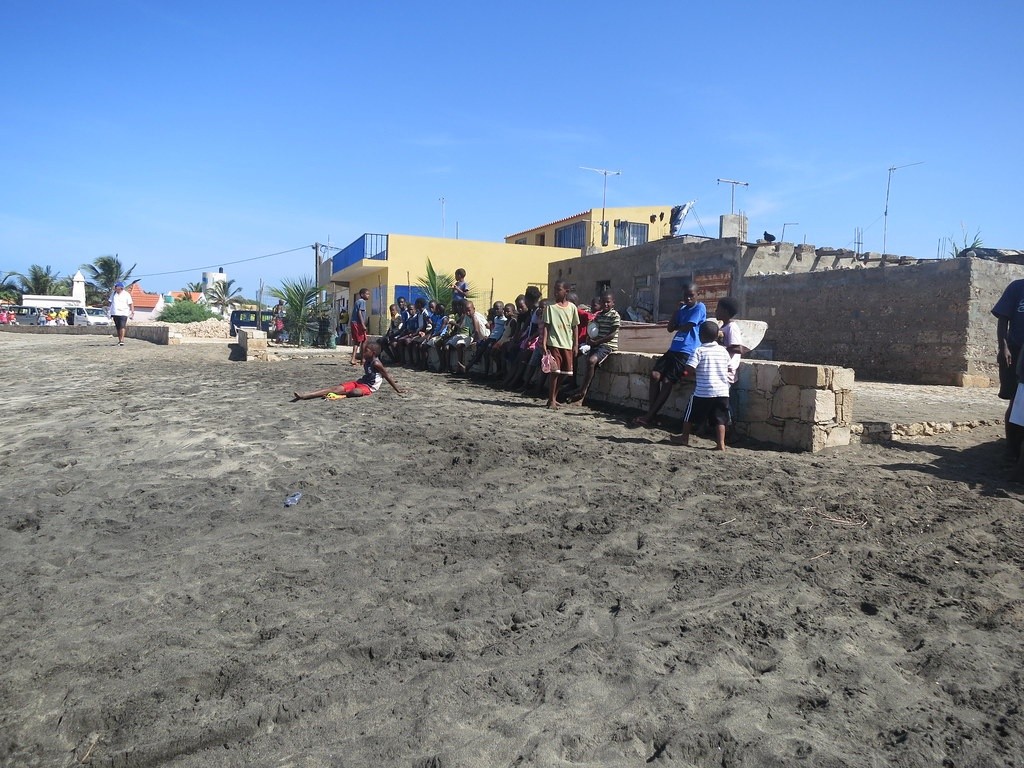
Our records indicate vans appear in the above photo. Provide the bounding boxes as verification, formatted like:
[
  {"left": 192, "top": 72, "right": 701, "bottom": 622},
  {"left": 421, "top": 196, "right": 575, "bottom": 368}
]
[
  {"left": 0, "top": 304, "right": 113, "bottom": 327},
  {"left": 230, "top": 310, "right": 287, "bottom": 338}
]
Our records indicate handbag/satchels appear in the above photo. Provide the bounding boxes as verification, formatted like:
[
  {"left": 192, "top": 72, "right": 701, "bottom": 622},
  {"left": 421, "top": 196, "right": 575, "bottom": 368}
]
[{"left": 106, "top": 304, "right": 112, "bottom": 320}]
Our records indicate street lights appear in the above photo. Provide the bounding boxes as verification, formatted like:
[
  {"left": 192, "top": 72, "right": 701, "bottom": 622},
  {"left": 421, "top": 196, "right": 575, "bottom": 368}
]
[{"left": 438, "top": 196, "right": 445, "bottom": 239}]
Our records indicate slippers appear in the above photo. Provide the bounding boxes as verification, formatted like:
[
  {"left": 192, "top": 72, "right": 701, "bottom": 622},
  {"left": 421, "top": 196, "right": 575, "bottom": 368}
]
[{"left": 541, "top": 349, "right": 558, "bottom": 374}]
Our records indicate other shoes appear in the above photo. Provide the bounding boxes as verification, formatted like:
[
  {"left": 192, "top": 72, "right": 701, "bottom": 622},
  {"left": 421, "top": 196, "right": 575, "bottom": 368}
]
[
  {"left": 120, "top": 341, "right": 124, "bottom": 344},
  {"left": 117, "top": 342, "right": 120, "bottom": 346}
]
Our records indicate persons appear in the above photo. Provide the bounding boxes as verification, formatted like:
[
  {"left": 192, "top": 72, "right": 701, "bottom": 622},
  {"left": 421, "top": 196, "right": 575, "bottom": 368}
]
[
  {"left": 0, "top": 305, "right": 17, "bottom": 325},
  {"left": 37, "top": 307, "right": 69, "bottom": 326},
  {"left": 632, "top": 282, "right": 707, "bottom": 427},
  {"left": 989, "top": 278, "right": 1024, "bottom": 485},
  {"left": 668, "top": 321, "right": 732, "bottom": 452},
  {"left": 350, "top": 288, "right": 370, "bottom": 365},
  {"left": 542, "top": 279, "right": 580, "bottom": 410},
  {"left": 292, "top": 342, "right": 406, "bottom": 399},
  {"left": 108, "top": 282, "right": 134, "bottom": 345},
  {"left": 271, "top": 317, "right": 288, "bottom": 343},
  {"left": 714, "top": 297, "right": 743, "bottom": 445},
  {"left": 374, "top": 268, "right": 620, "bottom": 411},
  {"left": 329, "top": 305, "right": 349, "bottom": 348}
]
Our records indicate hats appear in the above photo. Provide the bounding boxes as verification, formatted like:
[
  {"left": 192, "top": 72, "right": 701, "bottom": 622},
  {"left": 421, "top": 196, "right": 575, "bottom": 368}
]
[
  {"left": 587, "top": 323, "right": 599, "bottom": 339},
  {"left": 61, "top": 307, "right": 65, "bottom": 309},
  {"left": 115, "top": 282, "right": 124, "bottom": 287},
  {"left": 50, "top": 308, "right": 54, "bottom": 311}
]
